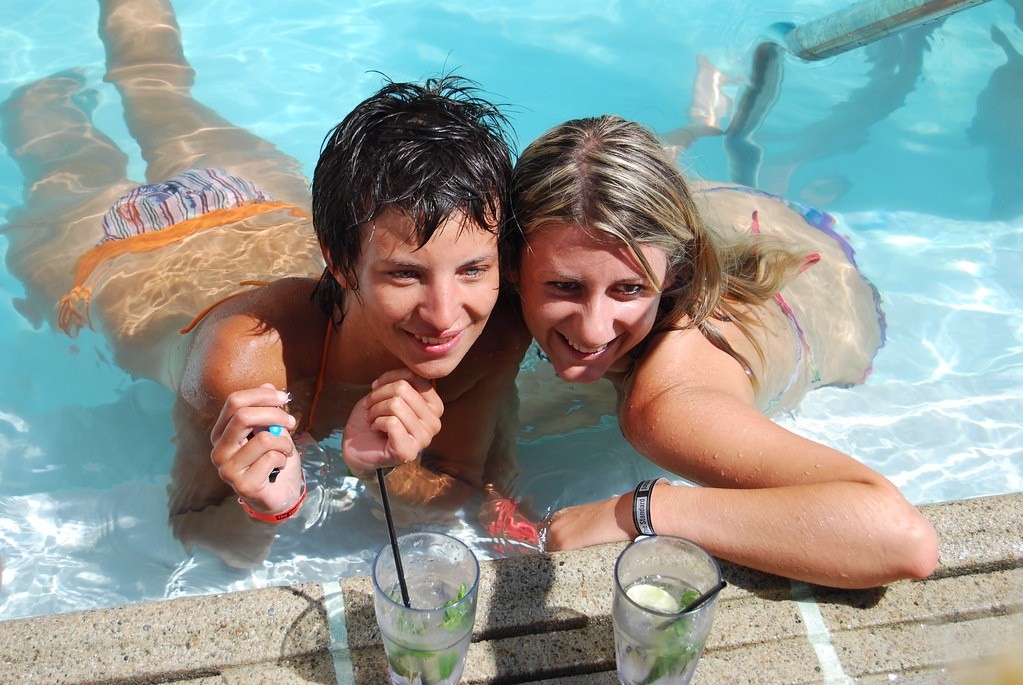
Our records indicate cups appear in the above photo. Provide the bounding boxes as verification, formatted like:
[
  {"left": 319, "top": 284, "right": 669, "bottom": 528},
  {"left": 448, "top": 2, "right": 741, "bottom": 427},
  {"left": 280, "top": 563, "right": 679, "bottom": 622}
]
[
  {"left": 612, "top": 536, "right": 723, "bottom": 685},
  {"left": 372, "top": 531, "right": 480, "bottom": 685}
]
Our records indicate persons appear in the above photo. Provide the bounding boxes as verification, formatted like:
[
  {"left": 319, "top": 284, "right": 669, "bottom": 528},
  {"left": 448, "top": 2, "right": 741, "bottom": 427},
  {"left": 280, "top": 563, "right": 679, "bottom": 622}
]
[
  {"left": 184, "top": 81, "right": 527, "bottom": 521},
  {"left": 511, "top": 115, "right": 939, "bottom": 589}
]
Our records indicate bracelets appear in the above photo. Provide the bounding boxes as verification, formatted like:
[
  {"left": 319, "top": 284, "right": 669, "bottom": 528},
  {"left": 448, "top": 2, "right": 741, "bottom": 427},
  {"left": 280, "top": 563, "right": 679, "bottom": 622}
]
[
  {"left": 238, "top": 467, "right": 307, "bottom": 524},
  {"left": 632, "top": 477, "right": 671, "bottom": 537},
  {"left": 374, "top": 467, "right": 396, "bottom": 483}
]
